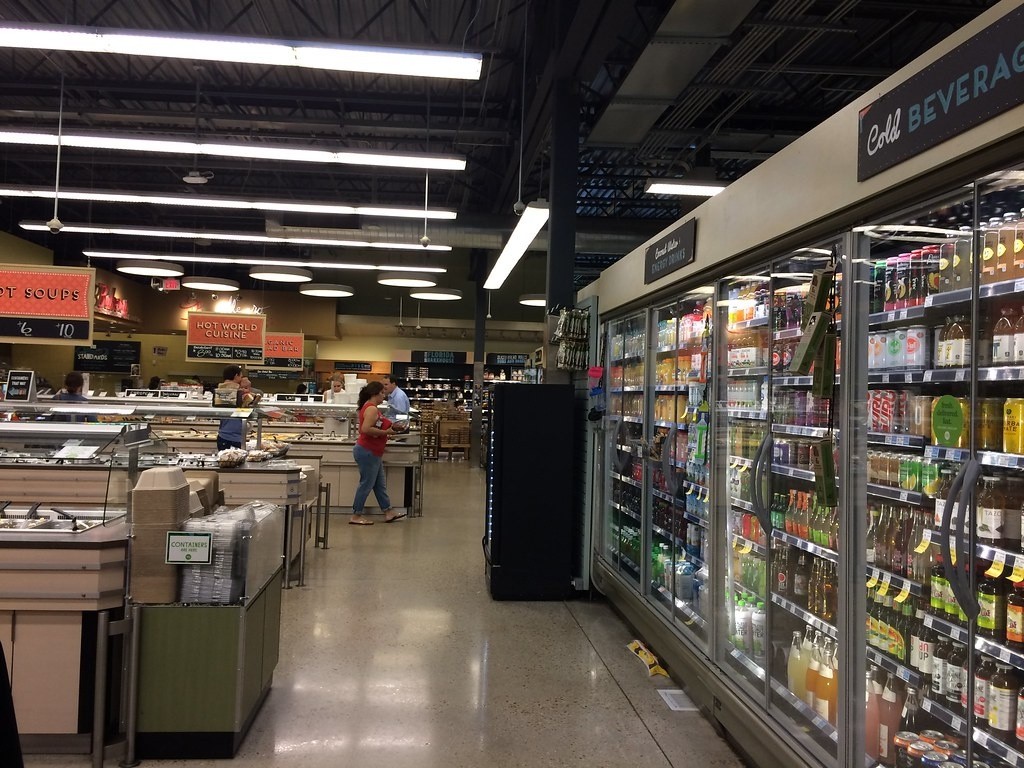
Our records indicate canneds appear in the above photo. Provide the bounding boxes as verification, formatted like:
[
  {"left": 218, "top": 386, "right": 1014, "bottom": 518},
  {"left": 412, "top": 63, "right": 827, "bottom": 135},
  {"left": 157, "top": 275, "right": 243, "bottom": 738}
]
[
  {"left": 870, "top": 243, "right": 938, "bottom": 313},
  {"left": 894, "top": 730, "right": 1009, "bottom": 768},
  {"left": 773, "top": 391, "right": 1024, "bottom": 453},
  {"left": 774, "top": 438, "right": 941, "bottom": 494},
  {"left": 867, "top": 324, "right": 931, "bottom": 371}
]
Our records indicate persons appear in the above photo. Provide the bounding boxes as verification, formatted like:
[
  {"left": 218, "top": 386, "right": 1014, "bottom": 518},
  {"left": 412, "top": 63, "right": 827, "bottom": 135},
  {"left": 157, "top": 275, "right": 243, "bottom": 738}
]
[
  {"left": 211, "top": 365, "right": 246, "bottom": 450},
  {"left": 192, "top": 375, "right": 204, "bottom": 386},
  {"left": 50, "top": 372, "right": 98, "bottom": 422},
  {"left": 146, "top": 376, "right": 163, "bottom": 397},
  {"left": 383, "top": 374, "right": 409, "bottom": 422},
  {"left": 349, "top": 381, "right": 408, "bottom": 525},
  {"left": 291, "top": 384, "right": 308, "bottom": 401},
  {"left": 322, "top": 372, "right": 350, "bottom": 434},
  {"left": 239, "top": 377, "right": 254, "bottom": 407}
]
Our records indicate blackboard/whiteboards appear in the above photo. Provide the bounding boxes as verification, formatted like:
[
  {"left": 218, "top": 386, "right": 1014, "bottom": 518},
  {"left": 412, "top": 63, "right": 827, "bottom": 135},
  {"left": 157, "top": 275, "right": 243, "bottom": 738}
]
[{"left": 73, "top": 339, "right": 142, "bottom": 374}]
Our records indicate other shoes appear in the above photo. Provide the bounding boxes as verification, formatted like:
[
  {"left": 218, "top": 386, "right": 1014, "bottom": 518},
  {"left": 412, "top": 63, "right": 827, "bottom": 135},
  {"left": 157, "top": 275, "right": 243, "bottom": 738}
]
[
  {"left": 384, "top": 512, "right": 406, "bottom": 523},
  {"left": 348, "top": 516, "right": 374, "bottom": 525}
]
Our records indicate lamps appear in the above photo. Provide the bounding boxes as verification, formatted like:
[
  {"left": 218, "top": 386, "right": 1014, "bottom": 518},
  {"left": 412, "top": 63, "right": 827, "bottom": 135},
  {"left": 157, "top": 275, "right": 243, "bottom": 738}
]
[
  {"left": 0, "top": 21, "right": 483, "bottom": 80},
  {"left": 0, "top": 122, "right": 467, "bottom": 171},
  {"left": 0, "top": 184, "right": 458, "bottom": 219},
  {"left": 115, "top": 255, "right": 547, "bottom": 307},
  {"left": 17, "top": 218, "right": 453, "bottom": 251},
  {"left": 483, "top": 198, "right": 550, "bottom": 289},
  {"left": 80, "top": 249, "right": 447, "bottom": 273},
  {"left": 643, "top": 178, "right": 732, "bottom": 197}
]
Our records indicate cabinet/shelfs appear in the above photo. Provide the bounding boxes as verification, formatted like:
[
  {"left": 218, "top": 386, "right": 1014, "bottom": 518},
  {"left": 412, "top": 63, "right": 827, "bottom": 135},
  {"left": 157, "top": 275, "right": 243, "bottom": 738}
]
[
  {"left": 420, "top": 419, "right": 440, "bottom": 462},
  {"left": 136, "top": 567, "right": 285, "bottom": 761},
  {"left": 397, "top": 377, "right": 521, "bottom": 411}
]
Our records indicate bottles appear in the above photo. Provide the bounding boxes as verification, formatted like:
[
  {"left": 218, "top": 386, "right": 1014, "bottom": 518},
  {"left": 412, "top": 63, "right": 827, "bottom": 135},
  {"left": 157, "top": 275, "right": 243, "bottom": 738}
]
[{"left": 601, "top": 208, "right": 1024, "bottom": 768}]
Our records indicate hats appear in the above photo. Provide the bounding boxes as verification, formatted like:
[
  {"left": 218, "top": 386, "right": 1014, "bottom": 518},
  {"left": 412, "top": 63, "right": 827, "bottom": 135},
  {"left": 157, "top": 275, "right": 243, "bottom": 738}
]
[{"left": 64, "top": 372, "right": 83, "bottom": 386}]
